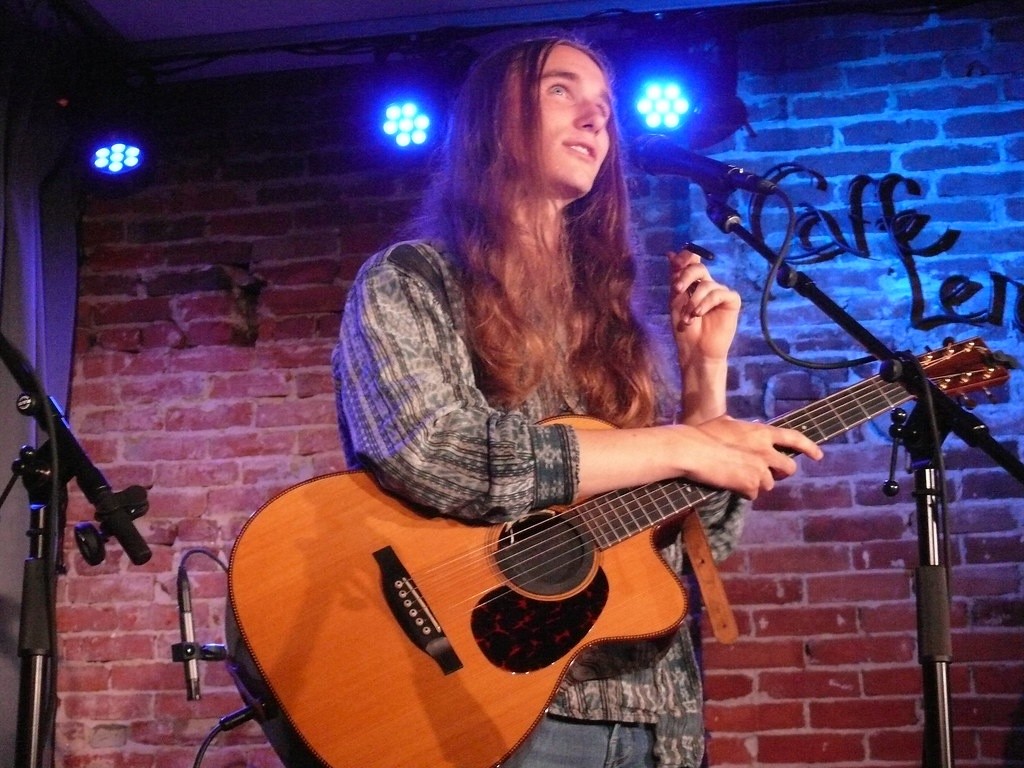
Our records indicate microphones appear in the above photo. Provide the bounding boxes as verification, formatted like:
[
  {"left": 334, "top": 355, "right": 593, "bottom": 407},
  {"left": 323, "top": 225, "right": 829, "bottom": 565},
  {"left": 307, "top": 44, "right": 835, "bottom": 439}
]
[
  {"left": 178, "top": 566, "right": 202, "bottom": 701},
  {"left": 630, "top": 134, "right": 778, "bottom": 193}
]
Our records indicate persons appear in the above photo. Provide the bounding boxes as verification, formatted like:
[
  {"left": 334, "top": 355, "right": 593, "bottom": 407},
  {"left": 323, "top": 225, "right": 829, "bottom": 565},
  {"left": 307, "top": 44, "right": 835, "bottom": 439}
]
[{"left": 331, "top": 31, "right": 822, "bottom": 768}]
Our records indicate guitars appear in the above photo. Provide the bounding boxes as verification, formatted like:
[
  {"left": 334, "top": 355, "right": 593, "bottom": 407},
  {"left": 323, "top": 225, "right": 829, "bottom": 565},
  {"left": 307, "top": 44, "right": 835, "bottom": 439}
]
[{"left": 220, "top": 331, "right": 1024, "bottom": 768}]
